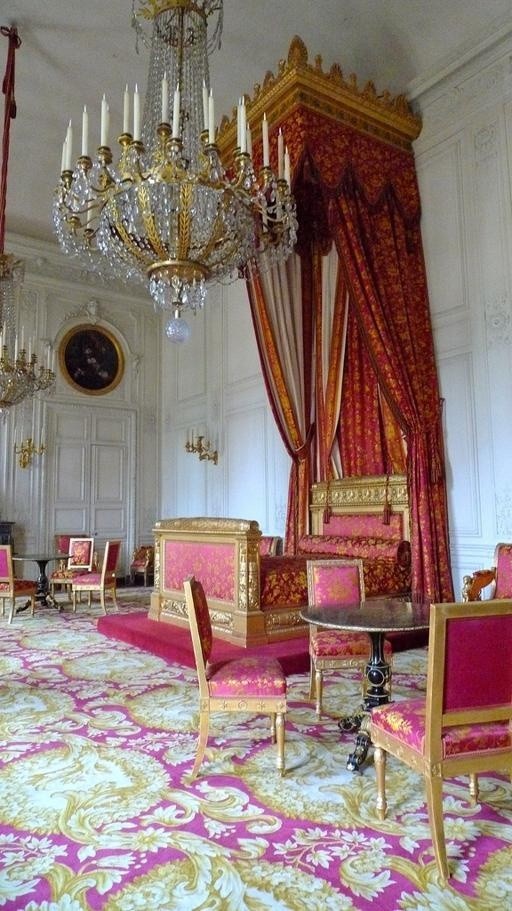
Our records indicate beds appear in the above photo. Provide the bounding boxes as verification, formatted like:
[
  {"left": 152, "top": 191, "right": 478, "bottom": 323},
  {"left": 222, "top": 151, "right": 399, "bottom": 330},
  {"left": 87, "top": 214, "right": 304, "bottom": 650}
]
[{"left": 146, "top": 473, "right": 414, "bottom": 644}]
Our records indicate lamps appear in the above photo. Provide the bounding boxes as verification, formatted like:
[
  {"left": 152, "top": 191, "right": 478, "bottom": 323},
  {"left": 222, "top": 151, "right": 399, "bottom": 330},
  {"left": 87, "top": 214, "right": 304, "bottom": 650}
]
[
  {"left": 179, "top": 425, "right": 220, "bottom": 465},
  {"left": 0, "top": 248, "right": 54, "bottom": 420},
  {"left": 11, "top": 424, "right": 47, "bottom": 473},
  {"left": 46, "top": 0, "right": 303, "bottom": 343}
]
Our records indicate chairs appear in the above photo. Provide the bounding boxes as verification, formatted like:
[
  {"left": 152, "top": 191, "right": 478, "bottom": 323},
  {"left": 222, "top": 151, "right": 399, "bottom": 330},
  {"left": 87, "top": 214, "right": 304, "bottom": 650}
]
[
  {"left": 181, "top": 576, "right": 291, "bottom": 777},
  {"left": 464, "top": 539, "right": 512, "bottom": 607},
  {"left": 2, "top": 531, "right": 155, "bottom": 627},
  {"left": 304, "top": 557, "right": 392, "bottom": 720},
  {"left": 369, "top": 595, "right": 511, "bottom": 883}
]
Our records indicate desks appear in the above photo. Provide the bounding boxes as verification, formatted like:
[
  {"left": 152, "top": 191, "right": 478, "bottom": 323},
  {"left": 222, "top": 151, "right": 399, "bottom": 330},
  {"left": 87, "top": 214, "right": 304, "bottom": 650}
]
[{"left": 294, "top": 600, "right": 446, "bottom": 771}]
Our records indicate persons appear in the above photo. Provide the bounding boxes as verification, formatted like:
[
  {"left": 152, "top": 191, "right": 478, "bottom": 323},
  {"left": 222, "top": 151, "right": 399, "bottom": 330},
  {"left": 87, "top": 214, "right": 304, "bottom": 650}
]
[{"left": 73, "top": 344, "right": 111, "bottom": 380}]
